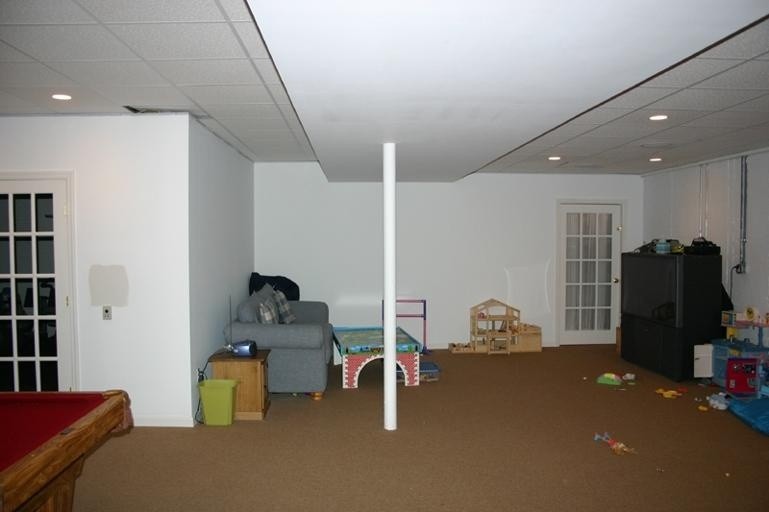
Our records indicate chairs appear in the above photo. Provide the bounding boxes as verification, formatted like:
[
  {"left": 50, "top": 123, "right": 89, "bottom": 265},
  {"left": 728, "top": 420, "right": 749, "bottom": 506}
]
[{"left": 222, "top": 300, "right": 333, "bottom": 402}]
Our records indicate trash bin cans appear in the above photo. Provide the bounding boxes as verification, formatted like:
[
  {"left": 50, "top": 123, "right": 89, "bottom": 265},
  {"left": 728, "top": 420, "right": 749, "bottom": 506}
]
[{"left": 196, "top": 379, "right": 236, "bottom": 426}]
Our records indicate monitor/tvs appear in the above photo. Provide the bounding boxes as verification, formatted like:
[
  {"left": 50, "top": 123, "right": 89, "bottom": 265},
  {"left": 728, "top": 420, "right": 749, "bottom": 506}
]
[{"left": 620, "top": 252, "right": 722, "bottom": 329}]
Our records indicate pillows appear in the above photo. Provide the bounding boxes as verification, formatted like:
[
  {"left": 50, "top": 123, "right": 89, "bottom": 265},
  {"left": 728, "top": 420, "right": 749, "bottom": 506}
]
[
  {"left": 258, "top": 295, "right": 280, "bottom": 324},
  {"left": 237, "top": 281, "right": 274, "bottom": 323},
  {"left": 272, "top": 290, "right": 298, "bottom": 325}
]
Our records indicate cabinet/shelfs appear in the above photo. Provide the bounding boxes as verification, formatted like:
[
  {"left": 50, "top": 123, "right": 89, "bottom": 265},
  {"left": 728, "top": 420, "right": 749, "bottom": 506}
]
[
  {"left": 620, "top": 314, "right": 723, "bottom": 383},
  {"left": 207, "top": 348, "right": 272, "bottom": 422}
]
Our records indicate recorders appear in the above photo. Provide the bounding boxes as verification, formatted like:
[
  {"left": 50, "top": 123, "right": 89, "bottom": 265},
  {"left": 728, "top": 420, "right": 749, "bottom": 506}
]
[{"left": 233, "top": 341, "right": 257, "bottom": 357}]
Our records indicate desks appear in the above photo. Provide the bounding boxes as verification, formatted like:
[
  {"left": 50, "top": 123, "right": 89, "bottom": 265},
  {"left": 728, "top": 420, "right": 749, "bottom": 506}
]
[
  {"left": 332, "top": 326, "right": 423, "bottom": 389},
  {"left": 0, "top": 388, "right": 135, "bottom": 512}
]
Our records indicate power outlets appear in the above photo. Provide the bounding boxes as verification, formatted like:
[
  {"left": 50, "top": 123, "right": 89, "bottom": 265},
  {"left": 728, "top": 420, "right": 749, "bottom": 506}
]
[{"left": 735, "top": 264, "right": 744, "bottom": 274}]
